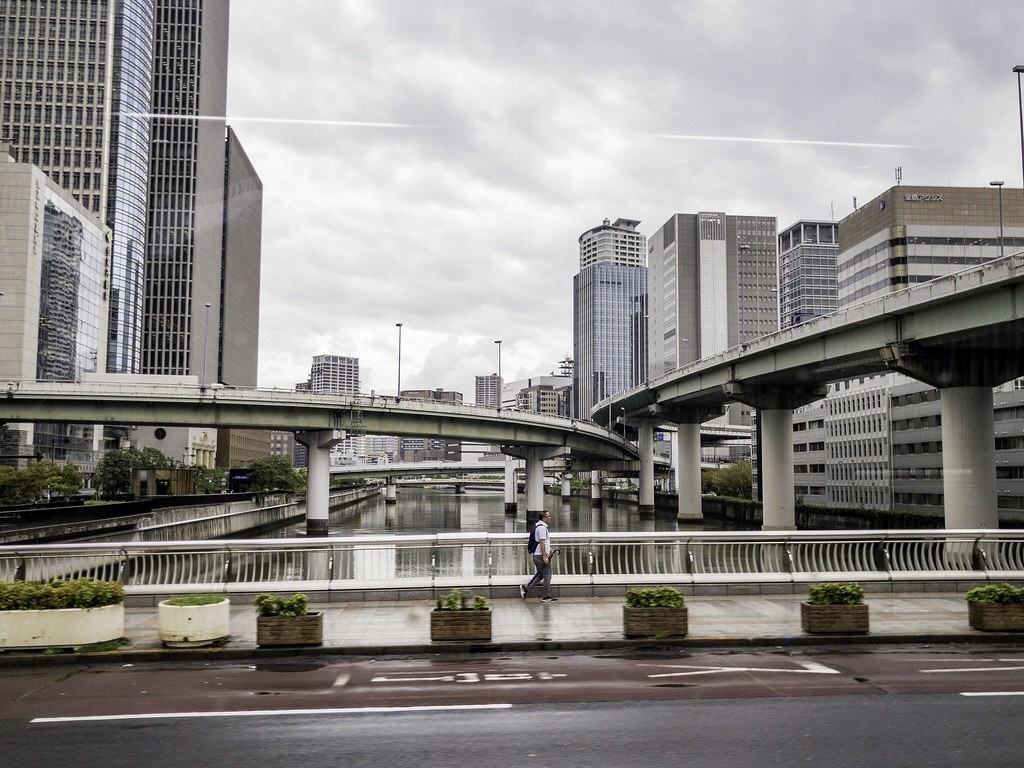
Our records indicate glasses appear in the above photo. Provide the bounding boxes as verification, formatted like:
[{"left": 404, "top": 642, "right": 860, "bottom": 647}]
[{"left": 547, "top": 515, "right": 551, "bottom": 517}]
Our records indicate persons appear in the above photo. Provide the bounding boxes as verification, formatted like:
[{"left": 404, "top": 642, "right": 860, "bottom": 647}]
[{"left": 520, "top": 511, "right": 558, "bottom": 602}]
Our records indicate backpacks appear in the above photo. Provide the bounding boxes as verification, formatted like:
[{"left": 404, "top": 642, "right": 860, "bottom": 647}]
[{"left": 527, "top": 524, "right": 547, "bottom": 554}]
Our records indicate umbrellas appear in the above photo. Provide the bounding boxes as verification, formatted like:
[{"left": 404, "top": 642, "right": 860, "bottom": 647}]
[{"left": 527, "top": 549, "right": 561, "bottom": 587}]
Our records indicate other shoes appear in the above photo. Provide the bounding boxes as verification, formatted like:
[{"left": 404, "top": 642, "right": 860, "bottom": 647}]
[
  {"left": 542, "top": 595, "right": 558, "bottom": 602},
  {"left": 520, "top": 585, "right": 528, "bottom": 600}
]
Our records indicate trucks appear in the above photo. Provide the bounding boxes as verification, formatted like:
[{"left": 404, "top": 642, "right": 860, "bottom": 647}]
[{"left": 229, "top": 468, "right": 255, "bottom": 492}]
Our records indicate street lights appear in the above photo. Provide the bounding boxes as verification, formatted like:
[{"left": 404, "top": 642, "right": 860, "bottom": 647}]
[
  {"left": 990, "top": 181, "right": 1004, "bottom": 257},
  {"left": 770, "top": 289, "right": 778, "bottom": 331},
  {"left": 621, "top": 407, "right": 626, "bottom": 438},
  {"left": 606, "top": 376, "right": 612, "bottom": 430},
  {"left": 396, "top": 323, "right": 403, "bottom": 399},
  {"left": 1013, "top": 64, "right": 1024, "bottom": 188},
  {"left": 203, "top": 303, "right": 211, "bottom": 384},
  {"left": 495, "top": 340, "right": 502, "bottom": 406},
  {"left": 682, "top": 339, "right": 688, "bottom": 364}
]
